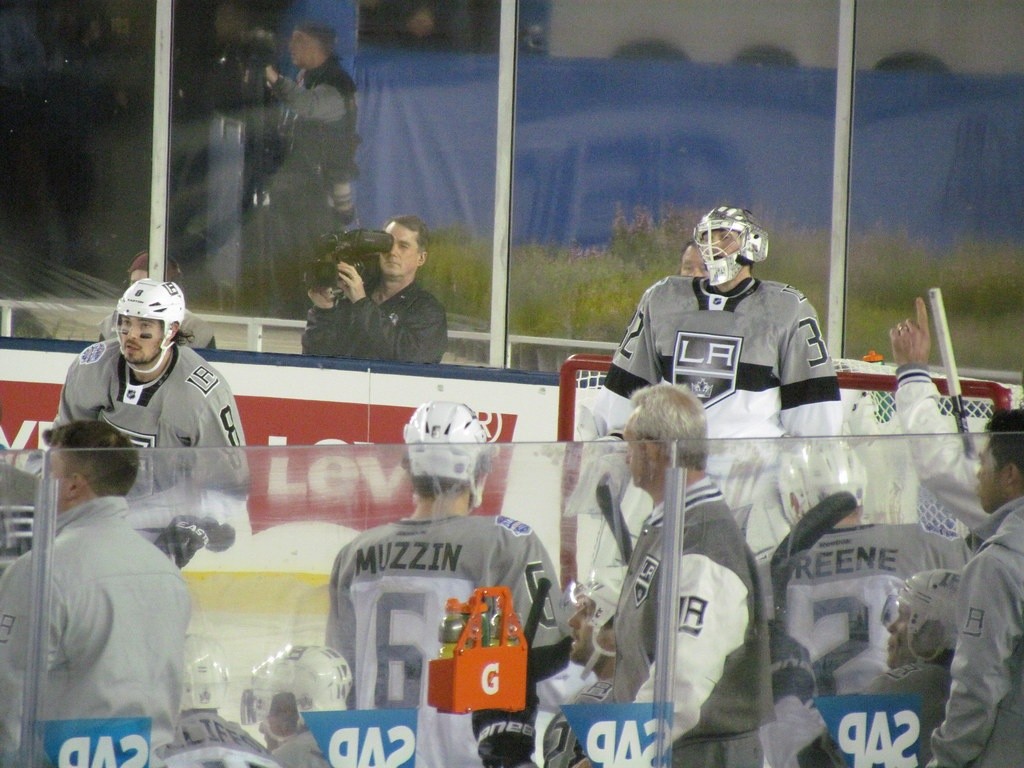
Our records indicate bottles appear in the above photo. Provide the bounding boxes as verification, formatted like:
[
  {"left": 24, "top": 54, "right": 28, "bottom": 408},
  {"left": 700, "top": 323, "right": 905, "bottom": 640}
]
[
  {"left": 864, "top": 351, "right": 889, "bottom": 423},
  {"left": 439, "top": 595, "right": 521, "bottom": 657}
]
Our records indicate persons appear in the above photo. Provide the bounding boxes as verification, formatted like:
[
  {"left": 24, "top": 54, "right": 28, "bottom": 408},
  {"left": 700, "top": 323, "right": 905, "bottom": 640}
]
[
  {"left": 564, "top": 206, "right": 867, "bottom": 657},
  {"left": 268, "top": 24, "right": 361, "bottom": 321},
  {"left": 0, "top": 421, "right": 189, "bottom": 768},
  {"left": 325, "top": 403, "right": 573, "bottom": 768},
  {"left": 543, "top": 383, "right": 777, "bottom": 768},
  {"left": 753, "top": 297, "right": 1024, "bottom": 768},
  {"left": 143, "top": 637, "right": 356, "bottom": 768},
  {"left": 302, "top": 213, "right": 449, "bottom": 365},
  {"left": 59, "top": 251, "right": 253, "bottom": 551}
]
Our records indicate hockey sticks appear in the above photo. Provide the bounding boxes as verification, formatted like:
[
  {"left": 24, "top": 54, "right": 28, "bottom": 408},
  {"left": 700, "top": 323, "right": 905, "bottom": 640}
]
[{"left": 927, "top": 285, "right": 980, "bottom": 463}]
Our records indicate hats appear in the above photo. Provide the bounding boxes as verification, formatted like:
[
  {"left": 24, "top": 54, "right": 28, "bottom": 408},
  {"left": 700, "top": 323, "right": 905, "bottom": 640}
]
[{"left": 132, "top": 252, "right": 181, "bottom": 284}]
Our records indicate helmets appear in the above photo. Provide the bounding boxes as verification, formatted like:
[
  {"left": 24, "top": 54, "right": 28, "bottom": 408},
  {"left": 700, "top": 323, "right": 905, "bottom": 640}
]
[
  {"left": 179, "top": 633, "right": 228, "bottom": 710},
  {"left": 115, "top": 279, "right": 185, "bottom": 337},
  {"left": 777, "top": 439, "right": 867, "bottom": 528},
  {"left": 402, "top": 401, "right": 488, "bottom": 481},
  {"left": 881, "top": 568, "right": 967, "bottom": 650},
  {"left": 248, "top": 643, "right": 352, "bottom": 728},
  {"left": 696, "top": 205, "right": 768, "bottom": 288},
  {"left": 578, "top": 562, "right": 630, "bottom": 631}
]
